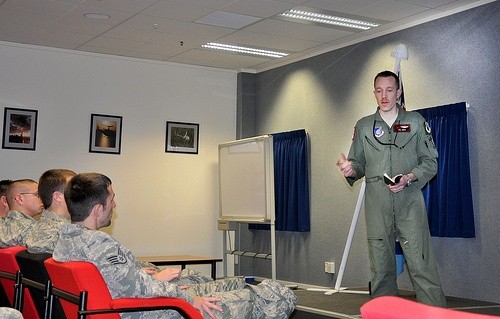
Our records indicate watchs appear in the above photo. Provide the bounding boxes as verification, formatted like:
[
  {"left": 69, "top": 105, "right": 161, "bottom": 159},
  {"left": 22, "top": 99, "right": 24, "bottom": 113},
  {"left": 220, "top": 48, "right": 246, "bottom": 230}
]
[{"left": 405, "top": 174, "right": 412, "bottom": 187}]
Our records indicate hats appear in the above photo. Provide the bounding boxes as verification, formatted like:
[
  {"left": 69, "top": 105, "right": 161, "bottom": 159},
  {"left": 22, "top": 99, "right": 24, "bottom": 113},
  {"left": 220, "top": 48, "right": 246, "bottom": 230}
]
[{"left": 244, "top": 278, "right": 299, "bottom": 318}]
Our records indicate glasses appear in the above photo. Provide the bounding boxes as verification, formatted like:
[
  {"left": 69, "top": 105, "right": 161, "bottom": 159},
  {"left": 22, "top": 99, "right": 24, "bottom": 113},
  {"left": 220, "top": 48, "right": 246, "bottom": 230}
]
[{"left": 15, "top": 191, "right": 39, "bottom": 200}]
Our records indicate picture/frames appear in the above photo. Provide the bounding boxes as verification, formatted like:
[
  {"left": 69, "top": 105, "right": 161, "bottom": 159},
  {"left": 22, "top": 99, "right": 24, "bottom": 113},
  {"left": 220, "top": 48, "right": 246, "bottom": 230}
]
[
  {"left": 165, "top": 121, "right": 200, "bottom": 154},
  {"left": 2, "top": 107, "right": 38, "bottom": 150},
  {"left": 88, "top": 114, "right": 123, "bottom": 154}
]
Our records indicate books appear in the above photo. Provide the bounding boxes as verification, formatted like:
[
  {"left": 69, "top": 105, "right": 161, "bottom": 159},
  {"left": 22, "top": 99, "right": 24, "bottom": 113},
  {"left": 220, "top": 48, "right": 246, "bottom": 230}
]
[{"left": 384, "top": 174, "right": 403, "bottom": 186}]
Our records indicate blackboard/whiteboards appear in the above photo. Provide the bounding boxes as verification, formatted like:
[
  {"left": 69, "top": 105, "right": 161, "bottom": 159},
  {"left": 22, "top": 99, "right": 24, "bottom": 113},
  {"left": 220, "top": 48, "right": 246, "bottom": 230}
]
[{"left": 217, "top": 134, "right": 271, "bottom": 224}]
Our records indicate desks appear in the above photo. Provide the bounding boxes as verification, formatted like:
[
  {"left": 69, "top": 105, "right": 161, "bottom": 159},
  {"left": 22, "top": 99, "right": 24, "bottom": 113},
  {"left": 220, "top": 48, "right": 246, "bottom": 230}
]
[{"left": 138, "top": 255, "right": 223, "bottom": 280}]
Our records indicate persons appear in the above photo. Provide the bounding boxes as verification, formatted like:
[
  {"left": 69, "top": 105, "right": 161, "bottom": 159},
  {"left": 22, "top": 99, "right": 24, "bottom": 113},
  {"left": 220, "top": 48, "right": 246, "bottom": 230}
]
[
  {"left": 0, "top": 169, "right": 297, "bottom": 319},
  {"left": 336, "top": 71, "right": 449, "bottom": 309}
]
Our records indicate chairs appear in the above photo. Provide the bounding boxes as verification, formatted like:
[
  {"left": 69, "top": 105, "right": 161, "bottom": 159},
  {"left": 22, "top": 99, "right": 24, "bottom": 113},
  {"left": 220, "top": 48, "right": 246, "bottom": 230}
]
[{"left": 0, "top": 246, "right": 205, "bottom": 319}]
